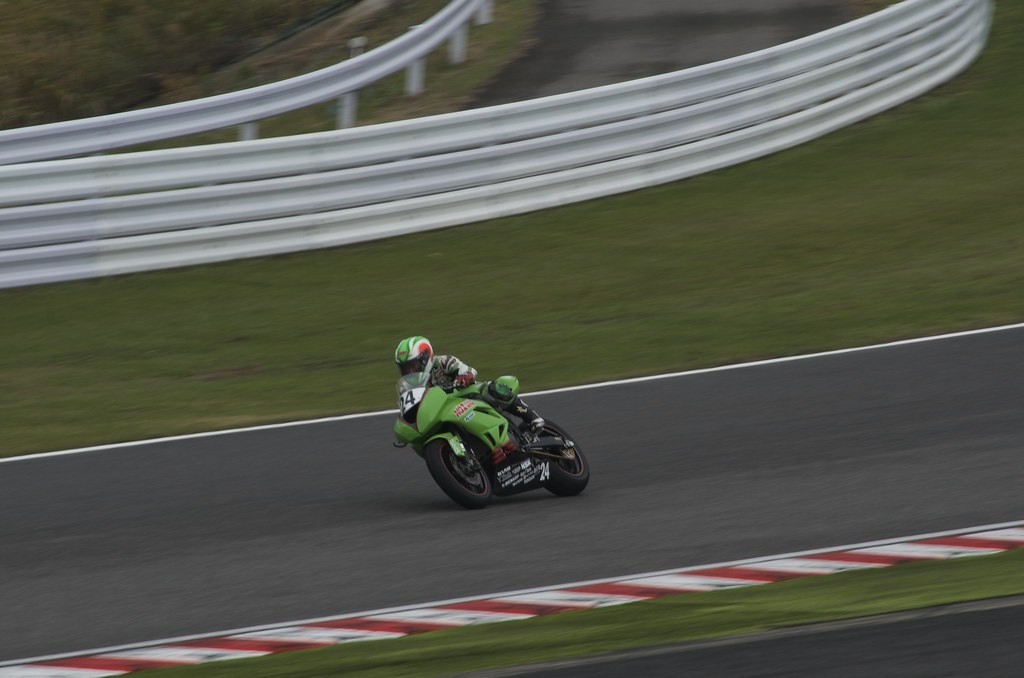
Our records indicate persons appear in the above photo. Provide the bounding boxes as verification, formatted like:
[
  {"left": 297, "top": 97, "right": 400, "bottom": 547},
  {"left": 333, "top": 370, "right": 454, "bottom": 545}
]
[{"left": 396, "top": 336, "right": 544, "bottom": 433}]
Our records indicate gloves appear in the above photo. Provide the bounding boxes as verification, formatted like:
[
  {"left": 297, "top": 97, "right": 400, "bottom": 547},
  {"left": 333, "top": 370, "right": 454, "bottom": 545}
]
[{"left": 453, "top": 372, "right": 473, "bottom": 388}]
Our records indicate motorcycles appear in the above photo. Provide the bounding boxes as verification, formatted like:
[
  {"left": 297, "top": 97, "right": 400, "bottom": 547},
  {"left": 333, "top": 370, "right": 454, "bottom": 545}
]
[{"left": 393, "top": 370, "right": 590, "bottom": 510}]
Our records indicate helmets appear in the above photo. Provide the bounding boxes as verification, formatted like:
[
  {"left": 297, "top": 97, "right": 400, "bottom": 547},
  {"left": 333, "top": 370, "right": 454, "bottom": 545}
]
[{"left": 394, "top": 336, "right": 433, "bottom": 386}]
